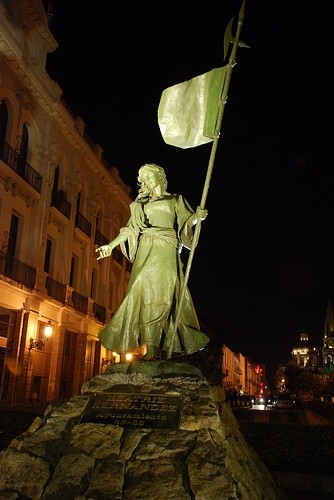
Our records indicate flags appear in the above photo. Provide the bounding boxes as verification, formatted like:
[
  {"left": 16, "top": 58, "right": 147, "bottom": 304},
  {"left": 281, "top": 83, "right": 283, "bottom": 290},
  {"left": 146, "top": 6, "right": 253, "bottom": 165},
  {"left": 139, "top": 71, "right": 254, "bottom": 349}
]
[{"left": 157, "top": 64, "right": 230, "bottom": 149}]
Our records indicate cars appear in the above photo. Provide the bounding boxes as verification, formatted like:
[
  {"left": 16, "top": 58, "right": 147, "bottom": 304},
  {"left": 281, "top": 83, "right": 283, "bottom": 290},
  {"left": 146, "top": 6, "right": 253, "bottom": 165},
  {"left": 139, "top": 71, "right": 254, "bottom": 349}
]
[{"left": 275, "top": 394, "right": 294, "bottom": 408}]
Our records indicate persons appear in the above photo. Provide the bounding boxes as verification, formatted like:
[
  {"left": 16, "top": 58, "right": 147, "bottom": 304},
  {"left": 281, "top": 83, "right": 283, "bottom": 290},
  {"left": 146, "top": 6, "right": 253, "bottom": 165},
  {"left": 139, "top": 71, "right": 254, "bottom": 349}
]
[{"left": 95, "top": 163, "right": 208, "bottom": 361}]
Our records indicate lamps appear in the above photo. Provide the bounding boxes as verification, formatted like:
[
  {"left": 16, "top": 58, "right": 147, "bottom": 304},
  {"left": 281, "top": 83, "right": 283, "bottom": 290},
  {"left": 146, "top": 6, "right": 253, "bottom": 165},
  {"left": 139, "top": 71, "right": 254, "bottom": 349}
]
[{"left": 43, "top": 320, "right": 54, "bottom": 338}]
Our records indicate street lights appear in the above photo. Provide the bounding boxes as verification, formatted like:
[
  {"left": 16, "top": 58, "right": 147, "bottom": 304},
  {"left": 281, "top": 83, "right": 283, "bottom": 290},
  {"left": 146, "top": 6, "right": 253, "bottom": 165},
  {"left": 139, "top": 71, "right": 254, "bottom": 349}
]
[
  {"left": 321, "top": 334, "right": 330, "bottom": 377},
  {"left": 311, "top": 347, "right": 319, "bottom": 372},
  {"left": 22, "top": 324, "right": 54, "bottom": 407}
]
